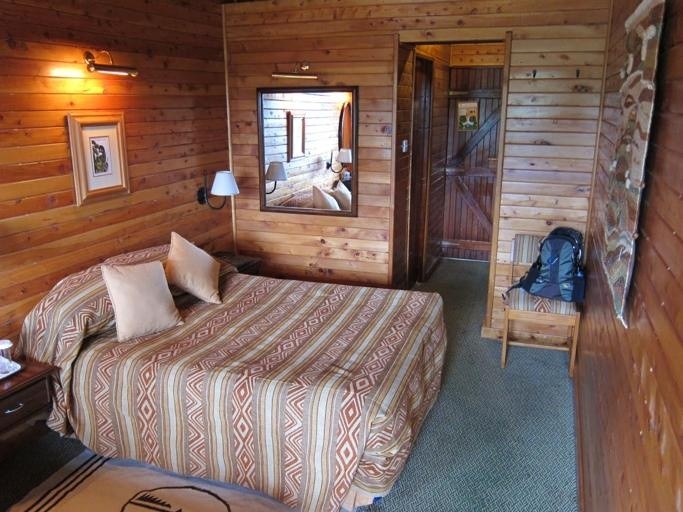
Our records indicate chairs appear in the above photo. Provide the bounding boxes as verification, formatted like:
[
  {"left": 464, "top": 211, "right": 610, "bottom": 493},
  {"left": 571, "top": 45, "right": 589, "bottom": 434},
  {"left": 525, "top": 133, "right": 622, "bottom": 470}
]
[{"left": 501, "top": 234, "right": 581, "bottom": 378}]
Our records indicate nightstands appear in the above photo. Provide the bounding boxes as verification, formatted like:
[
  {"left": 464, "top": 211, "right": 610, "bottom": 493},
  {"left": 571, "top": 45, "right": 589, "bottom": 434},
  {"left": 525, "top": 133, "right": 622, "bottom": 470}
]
[
  {"left": 0, "top": 360, "right": 55, "bottom": 464},
  {"left": 212, "top": 252, "right": 261, "bottom": 276}
]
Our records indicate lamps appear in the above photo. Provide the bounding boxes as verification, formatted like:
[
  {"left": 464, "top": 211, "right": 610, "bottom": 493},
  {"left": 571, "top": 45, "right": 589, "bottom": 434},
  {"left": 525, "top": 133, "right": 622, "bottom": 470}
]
[
  {"left": 197, "top": 168, "right": 240, "bottom": 210},
  {"left": 272, "top": 63, "right": 318, "bottom": 79},
  {"left": 265, "top": 162, "right": 286, "bottom": 194},
  {"left": 327, "top": 148, "right": 353, "bottom": 173},
  {"left": 85, "top": 50, "right": 139, "bottom": 77}
]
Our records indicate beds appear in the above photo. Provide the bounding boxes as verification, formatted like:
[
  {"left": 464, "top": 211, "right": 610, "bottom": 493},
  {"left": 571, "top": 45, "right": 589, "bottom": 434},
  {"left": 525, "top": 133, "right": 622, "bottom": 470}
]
[{"left": 12, "top": 244, "right": 447, "bottom": 512}]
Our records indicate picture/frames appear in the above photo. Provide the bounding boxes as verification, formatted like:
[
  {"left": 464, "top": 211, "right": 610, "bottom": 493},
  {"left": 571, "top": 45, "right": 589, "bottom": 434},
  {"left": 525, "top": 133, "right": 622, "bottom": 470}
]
[
  {"left": 287, "top": 111, "right": 305, "bottom": 162},
  {"left": 67, "top": 111, "right": 131, "bottom": 208}
]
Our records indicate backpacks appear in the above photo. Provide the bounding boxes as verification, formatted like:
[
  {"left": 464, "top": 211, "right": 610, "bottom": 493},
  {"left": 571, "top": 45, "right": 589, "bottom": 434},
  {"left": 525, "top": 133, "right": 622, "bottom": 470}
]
[{"left": 520, "top": 227, "right": 584, "bottom": 302}]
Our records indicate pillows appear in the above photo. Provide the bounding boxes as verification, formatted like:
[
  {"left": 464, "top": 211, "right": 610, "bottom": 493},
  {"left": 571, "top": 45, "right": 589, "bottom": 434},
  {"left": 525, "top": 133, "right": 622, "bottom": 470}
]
[
  {"left": 332, "top": 180, "right": 352, "bottom": 210},
  {"left": 101, "top": 260, "right": 185, "bottom": 342},
  {"left": 165, "top": 231, "right": 222, "bottom": 305},
  {"left": 312, "top": 187, "right": 341, "bottom": 210}
]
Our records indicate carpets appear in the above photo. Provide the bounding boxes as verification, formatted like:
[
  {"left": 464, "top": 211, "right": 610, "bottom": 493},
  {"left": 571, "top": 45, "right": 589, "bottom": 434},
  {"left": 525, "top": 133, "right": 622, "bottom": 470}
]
[{"left": 6, "top": 446, "right": 295, "bottom": 512}]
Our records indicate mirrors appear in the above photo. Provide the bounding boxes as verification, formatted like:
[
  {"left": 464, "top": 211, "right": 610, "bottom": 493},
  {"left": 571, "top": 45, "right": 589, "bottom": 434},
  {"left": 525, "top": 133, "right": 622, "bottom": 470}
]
[{"left": 256, "top": 86, "right": 358, "bottom": 216}]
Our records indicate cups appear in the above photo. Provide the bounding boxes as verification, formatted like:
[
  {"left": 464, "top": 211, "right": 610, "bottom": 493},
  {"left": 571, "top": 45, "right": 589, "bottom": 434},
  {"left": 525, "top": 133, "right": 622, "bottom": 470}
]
[{"left": 0, "top": 339, "right": 14, "bottom": 373}]
